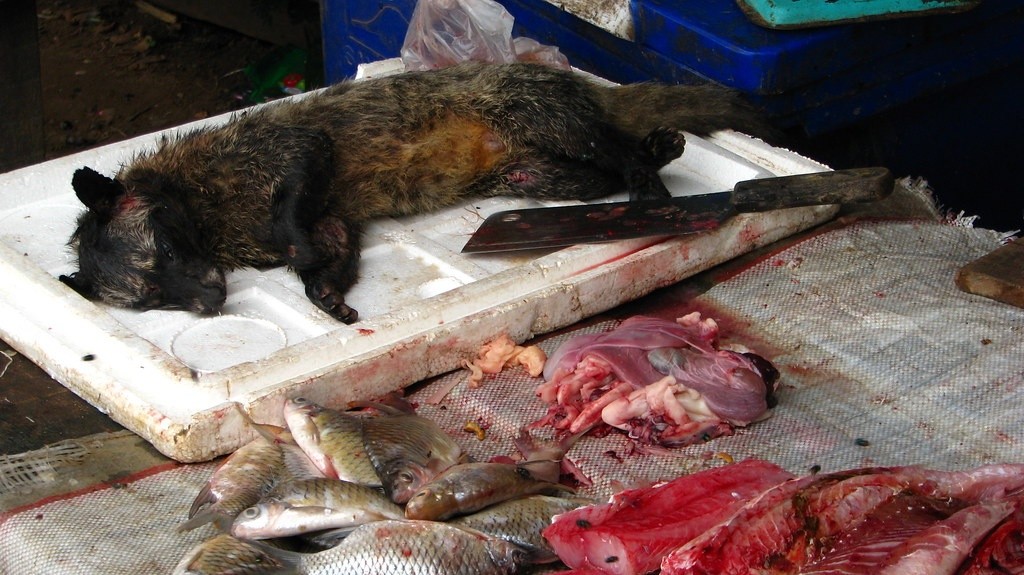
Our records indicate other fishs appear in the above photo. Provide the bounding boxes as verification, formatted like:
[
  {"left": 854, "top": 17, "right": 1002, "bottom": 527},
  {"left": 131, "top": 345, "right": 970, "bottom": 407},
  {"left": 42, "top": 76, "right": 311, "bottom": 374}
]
[{"left": 175, "top": 396, "right": 1024, "bottom": 574}]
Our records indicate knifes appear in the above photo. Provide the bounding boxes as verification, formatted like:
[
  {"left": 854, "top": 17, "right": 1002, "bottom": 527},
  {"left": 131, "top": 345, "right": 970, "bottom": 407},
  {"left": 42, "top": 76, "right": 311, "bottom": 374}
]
[{"left": 460, "top": 171, "right": 892, "bottom": 254}]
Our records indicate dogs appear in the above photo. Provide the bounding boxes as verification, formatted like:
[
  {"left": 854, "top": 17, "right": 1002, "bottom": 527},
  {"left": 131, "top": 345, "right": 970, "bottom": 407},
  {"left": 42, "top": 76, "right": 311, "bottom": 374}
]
[{"left": 59, "top": 62, "right": 755, "bottom": 324}]
